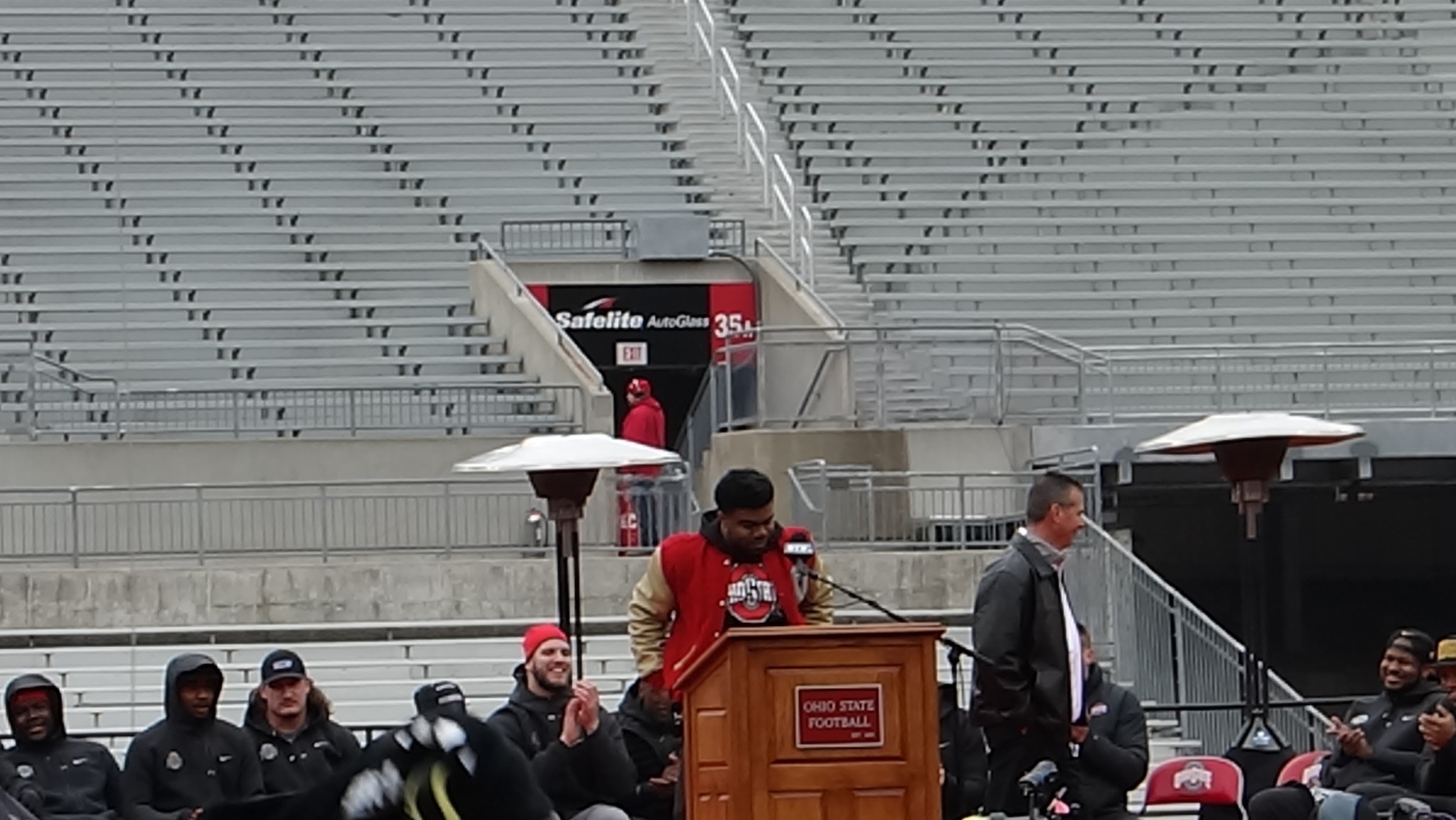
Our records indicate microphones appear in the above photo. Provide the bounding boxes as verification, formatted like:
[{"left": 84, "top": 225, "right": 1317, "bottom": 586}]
[{"left": 782, "top": 532, "right": 815, "bottom": 574}]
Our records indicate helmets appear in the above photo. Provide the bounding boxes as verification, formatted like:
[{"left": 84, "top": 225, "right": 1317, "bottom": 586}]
[{"left": 626, "top": 378, "right": 650, "bottom": 397}]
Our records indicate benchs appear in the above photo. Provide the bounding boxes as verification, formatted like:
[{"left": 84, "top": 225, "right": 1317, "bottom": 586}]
[{"left": 2, "top": 0, "right": 1456, "bottom": 768}]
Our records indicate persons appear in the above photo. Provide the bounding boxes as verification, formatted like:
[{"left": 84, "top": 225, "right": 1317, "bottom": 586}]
[
  {"left": 628, "top": 468, "right": 834, "bottom": 714},
  {"left": 1247, "top": 629, "right": 1456, "bottom": 820},
  {"left": 241, "top": 649, "right": 362, "bottom": 820},
  {"left": 124, "top": 654, "right": 270, "bottom": 820},
  {"left": 340, "top": 622, "right": 682, "bottom": 820},
  {"left": 967, "top": 473, "right": 1085, "bottom": 814},
  {"left": 939, "top": 624, "right": 1150, "bottom": 820},
  {"left": 0, "top": 673, "right": 122, "bottom": 820},
  {"left": 618, "top": 378, "right": 666, "bottom": 557}
]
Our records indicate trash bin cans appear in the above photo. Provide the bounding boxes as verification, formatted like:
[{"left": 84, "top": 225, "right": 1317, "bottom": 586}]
[{"left": 617, "top": 489, "right": 638, "bottom": 547}]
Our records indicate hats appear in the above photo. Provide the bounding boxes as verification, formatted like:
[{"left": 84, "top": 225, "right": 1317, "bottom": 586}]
[
  {"left": 523, "top": 623, "right": 567, "bottom": 661},
  {"left": 1437, "top": 635, "right": 1456, "bottom": 665},
  {"left": 329, "top": 680, "right": 555, "bottom": 820},
  {"left": 7, "top": 691, "right": 49, "bottom": 712},
  {"left": 261, "top": 651, "right": 306, "bottom": 685},
  {"left": 1388, "top": 630, "right": 1435, "bottom": 664}
]
